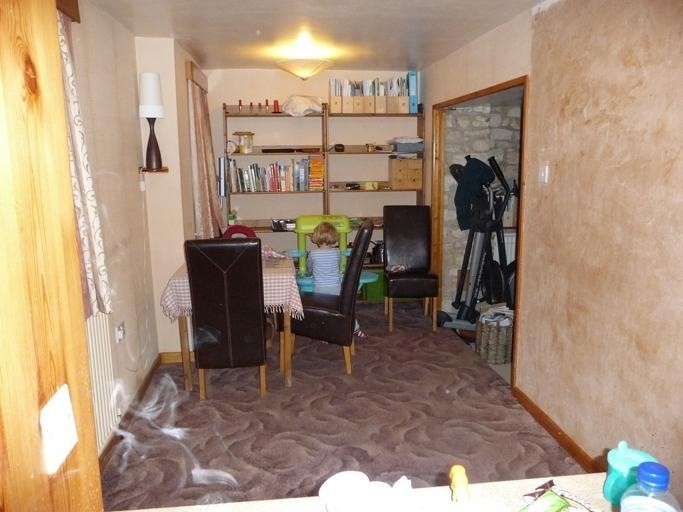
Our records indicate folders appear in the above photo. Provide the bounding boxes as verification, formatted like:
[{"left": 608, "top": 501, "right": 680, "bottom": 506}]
[{"left": 408, "top": 71, "right": 418, "bottom": 113}]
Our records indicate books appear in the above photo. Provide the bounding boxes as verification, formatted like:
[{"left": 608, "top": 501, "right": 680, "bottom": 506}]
[{"left": 230, "top": 157, "right": 325, "bottom": 191}]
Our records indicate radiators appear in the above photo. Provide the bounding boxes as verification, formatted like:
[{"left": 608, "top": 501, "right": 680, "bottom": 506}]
[{"left": 87, "top": 306, "right": 122, "bottom": 458}]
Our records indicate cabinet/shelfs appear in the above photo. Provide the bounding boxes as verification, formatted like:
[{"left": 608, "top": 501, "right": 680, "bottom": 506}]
[{"left": 221, "top": 99, "right": 425, "bottom": 238}]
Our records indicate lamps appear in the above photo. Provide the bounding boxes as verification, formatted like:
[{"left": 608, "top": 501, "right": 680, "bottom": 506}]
[
  {"left": 136, "top": 71, "right": 167, "bottom": 170},
  {"left": 277, "top": 56, "right": 333, "bottom": 81}
]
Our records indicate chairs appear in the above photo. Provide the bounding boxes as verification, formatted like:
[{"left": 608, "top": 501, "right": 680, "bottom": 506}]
[
  {"left": 382, "top": 204, "right": 440, "bottom": 333},
  {"left": 184, "top": 222, "right": 388, "bottom": 399}
]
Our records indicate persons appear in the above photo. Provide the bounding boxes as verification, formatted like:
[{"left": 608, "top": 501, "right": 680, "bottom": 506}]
[{"left": 306, "top": 221, "right": 369, "bottom": 339}]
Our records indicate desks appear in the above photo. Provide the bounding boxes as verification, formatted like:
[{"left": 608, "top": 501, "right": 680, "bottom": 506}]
[{"left": 167, "top": 253, "right": 297, "bottom": 393}]
